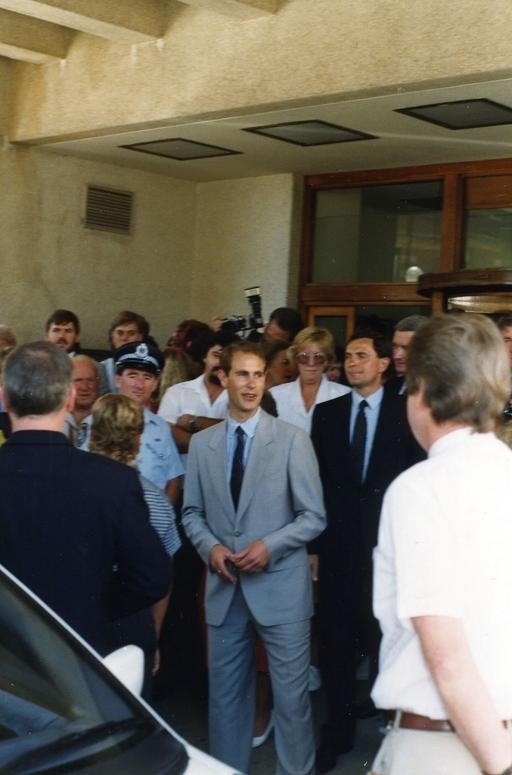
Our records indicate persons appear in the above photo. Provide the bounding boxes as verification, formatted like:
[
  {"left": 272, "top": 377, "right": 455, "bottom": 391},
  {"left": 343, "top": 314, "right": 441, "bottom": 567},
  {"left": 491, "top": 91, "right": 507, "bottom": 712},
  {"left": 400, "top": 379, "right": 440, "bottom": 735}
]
[{"left": 0, "top": 305, "right": 511, "bottom": 775}]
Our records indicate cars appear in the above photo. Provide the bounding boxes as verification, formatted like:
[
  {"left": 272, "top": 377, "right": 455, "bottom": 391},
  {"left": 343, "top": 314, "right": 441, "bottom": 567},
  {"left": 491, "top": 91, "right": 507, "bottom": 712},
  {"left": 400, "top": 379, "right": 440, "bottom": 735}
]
[{"left": 0, "top": 563, "right": 244, "bottom": 775}]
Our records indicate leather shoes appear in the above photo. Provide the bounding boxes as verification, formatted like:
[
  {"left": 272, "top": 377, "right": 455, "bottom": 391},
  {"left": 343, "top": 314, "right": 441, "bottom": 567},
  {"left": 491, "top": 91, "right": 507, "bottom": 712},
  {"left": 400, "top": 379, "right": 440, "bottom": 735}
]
[{"left": 316, "top": 725, "right": 354, "bottom": 772}]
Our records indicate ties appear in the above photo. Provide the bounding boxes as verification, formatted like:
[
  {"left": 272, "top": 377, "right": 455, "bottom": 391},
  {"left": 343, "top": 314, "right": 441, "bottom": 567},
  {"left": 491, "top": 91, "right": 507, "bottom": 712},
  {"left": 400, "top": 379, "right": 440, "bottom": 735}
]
[
  {"left": 353, "top": 400, "right": 368, "bottom": 485},
  {"left": 231, "top": 426, "right": 244, "bottom": 512}
]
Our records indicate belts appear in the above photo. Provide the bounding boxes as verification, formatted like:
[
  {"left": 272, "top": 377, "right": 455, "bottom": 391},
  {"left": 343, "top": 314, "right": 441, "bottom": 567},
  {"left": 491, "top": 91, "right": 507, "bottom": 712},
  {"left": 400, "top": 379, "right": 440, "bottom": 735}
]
[{"left": 390, "top": 713, "right": 511, "bottom": 732}]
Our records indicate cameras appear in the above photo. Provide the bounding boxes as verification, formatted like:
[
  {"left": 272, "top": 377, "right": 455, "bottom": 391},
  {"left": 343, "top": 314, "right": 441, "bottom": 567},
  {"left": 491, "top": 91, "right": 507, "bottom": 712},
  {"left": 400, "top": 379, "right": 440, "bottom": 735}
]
[
  {"left": 244, "top": 286, "right": 264, "bottom": 330},
  {"left": 218, "top": 314, "right": 246, "bottom": 334}
]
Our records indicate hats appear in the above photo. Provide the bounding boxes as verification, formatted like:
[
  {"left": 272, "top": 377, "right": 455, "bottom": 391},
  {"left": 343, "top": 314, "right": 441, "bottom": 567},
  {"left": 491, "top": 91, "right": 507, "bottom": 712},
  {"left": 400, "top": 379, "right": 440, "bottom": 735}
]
[{"left": 114, "top": 341, "right": 164, "bottom": 374}]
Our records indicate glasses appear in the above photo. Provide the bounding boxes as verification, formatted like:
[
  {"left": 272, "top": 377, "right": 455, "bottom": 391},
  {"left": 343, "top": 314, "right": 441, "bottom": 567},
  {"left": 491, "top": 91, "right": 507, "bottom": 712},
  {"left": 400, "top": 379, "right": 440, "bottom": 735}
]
[{"left": 296, "top": 352, "right": 327, "bottom": 365}]
